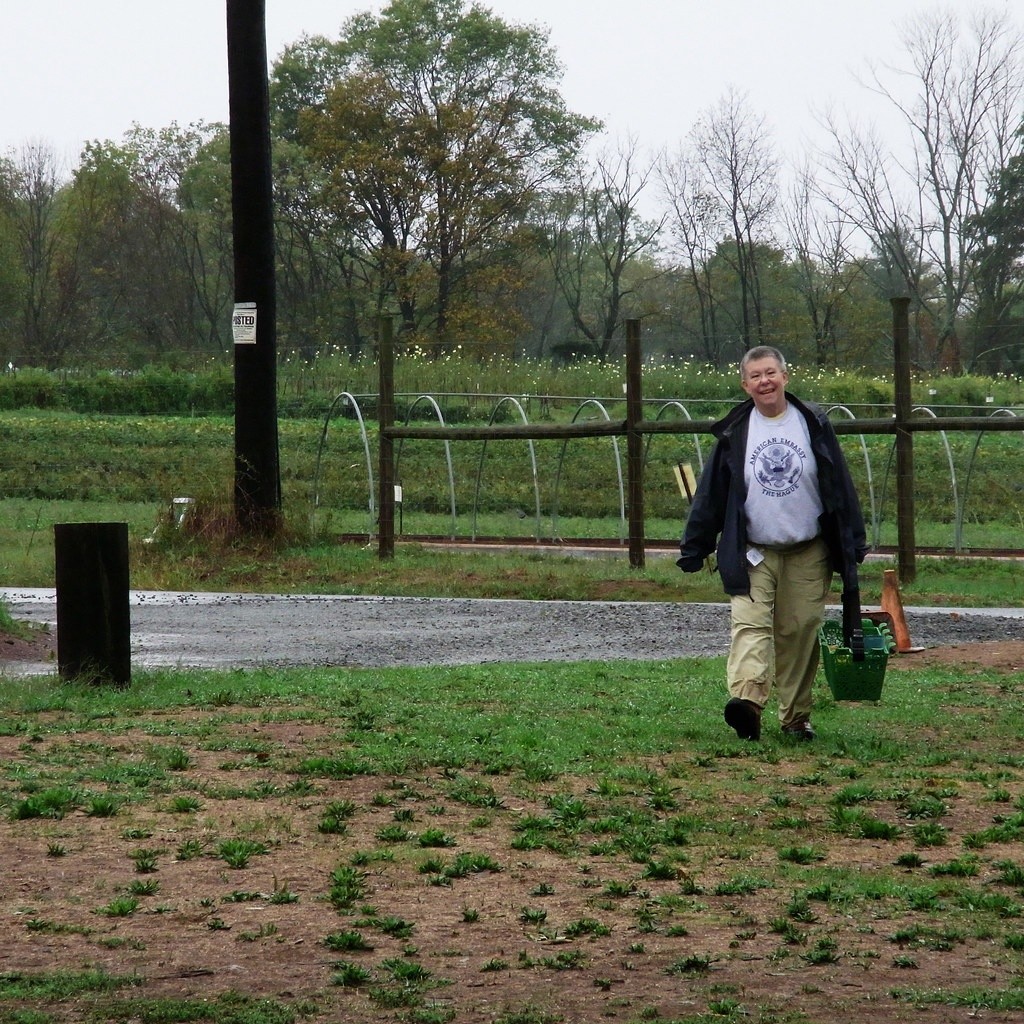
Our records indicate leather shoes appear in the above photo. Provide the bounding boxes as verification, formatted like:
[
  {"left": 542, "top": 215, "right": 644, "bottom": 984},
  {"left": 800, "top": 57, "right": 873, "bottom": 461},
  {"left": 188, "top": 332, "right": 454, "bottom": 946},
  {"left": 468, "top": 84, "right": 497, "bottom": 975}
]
[
  {"left": 725, "top": 697, "right": 760, "bottom": 740},
  {"left": 784, "top": 721, "right": 817, "bottom": 742}
]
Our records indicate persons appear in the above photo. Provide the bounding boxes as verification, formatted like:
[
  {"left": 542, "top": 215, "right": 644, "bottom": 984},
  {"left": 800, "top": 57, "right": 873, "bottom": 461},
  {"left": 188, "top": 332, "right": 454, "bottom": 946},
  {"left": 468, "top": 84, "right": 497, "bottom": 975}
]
[{"left": 675, "top": 346, "right": 866, "bottom": 745}]
